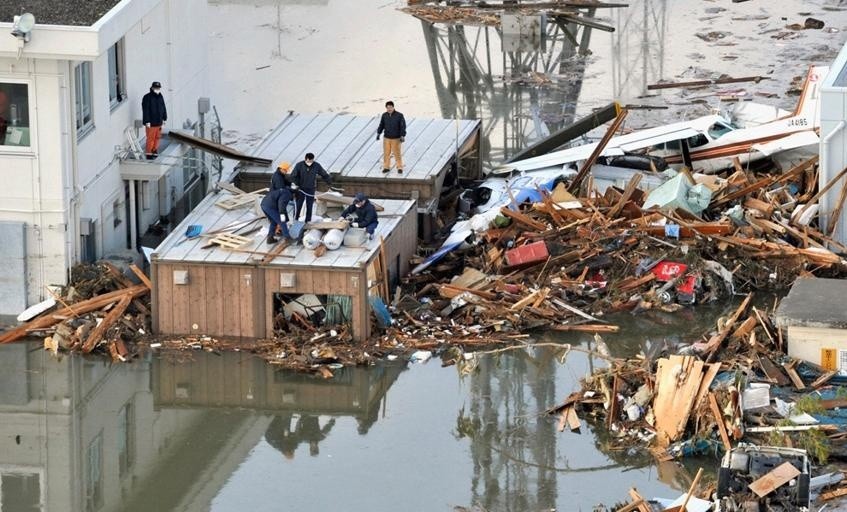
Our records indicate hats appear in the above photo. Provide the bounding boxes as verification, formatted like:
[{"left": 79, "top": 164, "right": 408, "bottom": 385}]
[
  {"left": 354, "top": 192, "right": 365, "bottom": 202},
  {"left": 152, "top": 81, "right": 161, "bottom": 89}
]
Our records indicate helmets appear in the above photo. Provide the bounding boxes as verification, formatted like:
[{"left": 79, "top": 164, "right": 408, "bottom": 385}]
[{"left": 279, "top": 162, "right": 290, "bottom": 173}]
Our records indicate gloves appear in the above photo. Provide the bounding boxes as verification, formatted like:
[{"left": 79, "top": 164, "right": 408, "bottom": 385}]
[
  {"left": 400, "top": 136, "right": 404, "bottom": 142},
  {"left": 163, "top": 121, "right": 168, "bottom": 126},
  {"left": 377, "top": 134, "right": 380, "bottom": 140},
  {"left": 145, "top": 122, "right": 151, "bottom": 129},
  {"left": 280, "top": 214, "right": 287, "bottom": 222}
]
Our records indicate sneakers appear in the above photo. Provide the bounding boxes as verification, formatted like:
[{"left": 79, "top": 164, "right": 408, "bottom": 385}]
[
  {"left": 369, "top": 234, "right": 375, "bottom": 240},
  {"left": 294, "top": 219, "right": 314, "bottom": 226},
  {"left": 380, "top": 169, "right": 402, "bottom": 173},
  {"left": 276, "top": 229, "right": 284, "bottom": 236},
  {"left": 145, "top": 151, "right": 158, "bottom": 159},
  {"left": 267, "top": 237, "right": 278, "bottom": 244}
]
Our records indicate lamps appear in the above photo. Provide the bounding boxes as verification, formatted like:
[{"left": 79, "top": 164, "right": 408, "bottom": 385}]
[{"left": 10, "top": 9, "right": 37, "bottom": 56}]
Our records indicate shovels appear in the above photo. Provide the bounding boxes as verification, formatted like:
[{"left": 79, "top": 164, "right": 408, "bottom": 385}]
[{"left": 177, "top": 225, "right": 202, "bottom": 246}]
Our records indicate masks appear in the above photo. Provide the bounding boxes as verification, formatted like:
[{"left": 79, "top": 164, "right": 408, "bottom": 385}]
[
  {"left": 305, "top": 161, "right": 313, "bottom": 166},
  {"left": 356, "top": 204, "right": 361, "bottom": 208},
  {"left": 155, "top": 90, "right": 161, "bottom": 93}
]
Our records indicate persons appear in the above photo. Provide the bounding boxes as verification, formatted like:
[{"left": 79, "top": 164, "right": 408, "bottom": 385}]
[
  {"left": 296, "top": 415, "right": 338, "bottom": 459},
  {"left": 354, "top": 402, "right": 381, "bottom": 436},
  {"left": 289, "top": 151, "right": 333, "bottom": 226},
  {"left": 339, "top": 193, "right": 379, "bottom": 241},
  {"left": 259, "top": 186, "right": 296, "bottom": 245},
  {"left": 263, "top": 414, "right": 297, "bottom": 462},
  {"left": 270, "top": 160, "right": 296, "bottom": 194},
  {"left": 374, "top": 99, "right": 405, "bottom": 174},
  {"left": 139, "top": 81, "right": 168, "bottom": 160}
]
